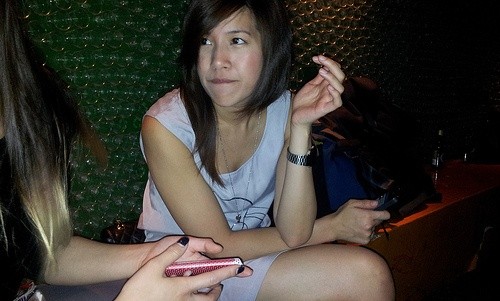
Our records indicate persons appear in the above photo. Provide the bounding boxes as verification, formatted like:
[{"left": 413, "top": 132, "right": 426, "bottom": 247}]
[
  {"left": 0, "top": 0, "right": 253, "bottom": 301},
  {"left": 137, "top": 0, "right": 395, "bottom": 301}
]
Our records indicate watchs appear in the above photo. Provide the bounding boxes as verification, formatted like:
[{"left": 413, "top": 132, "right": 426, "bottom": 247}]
[{"left": 286, "top": 147, "right": 320, "bottom": 168}]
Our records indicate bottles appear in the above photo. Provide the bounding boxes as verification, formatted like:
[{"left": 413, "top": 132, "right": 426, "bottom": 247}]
[{"left": 431, "top": 128, "right": 445, "bottom": 169}]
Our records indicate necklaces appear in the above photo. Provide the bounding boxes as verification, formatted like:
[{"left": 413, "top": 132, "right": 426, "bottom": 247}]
[{"left": 213, "top": 109, "right": 261, "bottom": 226}]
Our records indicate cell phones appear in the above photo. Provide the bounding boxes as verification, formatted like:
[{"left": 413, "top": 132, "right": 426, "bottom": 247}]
[
  {"left": 164, "top": 258, "right": 243, "bottom": 278},
  {"left": 372, "top": 188, "right": 401, "bottom": 211}
]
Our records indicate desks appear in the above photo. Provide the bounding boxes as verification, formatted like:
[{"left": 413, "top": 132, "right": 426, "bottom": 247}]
[{"left": 326, "top": 163, "right": 500, "bottom": 301}]
